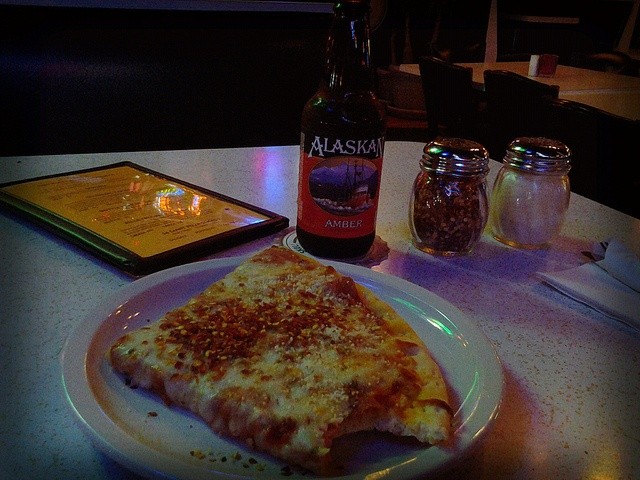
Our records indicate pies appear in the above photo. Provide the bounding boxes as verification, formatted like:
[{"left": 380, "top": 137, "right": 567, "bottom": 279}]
[{"left": 109, "top": 245, "right": 453, "bottom": 479}]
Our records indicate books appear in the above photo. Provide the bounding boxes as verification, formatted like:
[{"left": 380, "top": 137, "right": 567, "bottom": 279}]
[{"left": 0, "top": 161, "right": 290, "bottom": 280}]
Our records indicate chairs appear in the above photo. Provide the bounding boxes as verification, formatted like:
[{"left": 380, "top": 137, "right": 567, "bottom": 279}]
[
  {"left": 419, "top": 56, "right": 474, "bottom": 144},
  {"left": 483, "top": 70, "right": 561, "bottom": 145}
]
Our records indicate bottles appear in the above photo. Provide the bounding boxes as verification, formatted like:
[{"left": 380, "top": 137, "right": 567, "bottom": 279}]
[
  {"left": 488, "top": 137, "right": 573, "bottom": 250},
  {"left": 408, "top": 135, "right": 490, "bottom": 258},
  {"left": 295, "top": 1, "right": 386, "bottom": 261}
]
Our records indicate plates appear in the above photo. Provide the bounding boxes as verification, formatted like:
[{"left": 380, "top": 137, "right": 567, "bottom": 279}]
[{"left": 59, "top": 252, "right": 503, "bottom": 480}]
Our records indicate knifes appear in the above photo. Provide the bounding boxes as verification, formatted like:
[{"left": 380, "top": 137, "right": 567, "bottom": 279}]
[{"left": 580, "top": 250, "right": 603, "bottom": 263}]
